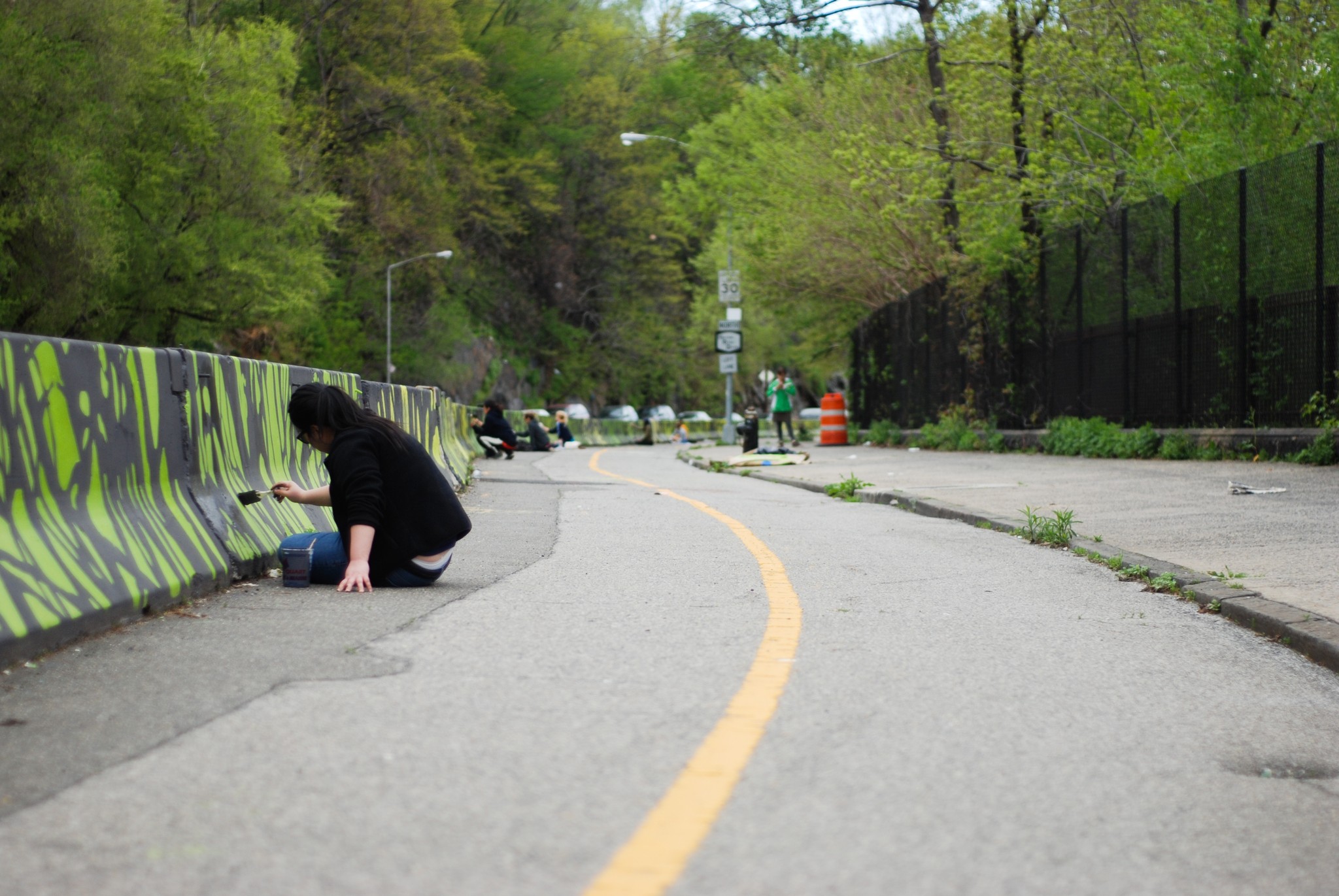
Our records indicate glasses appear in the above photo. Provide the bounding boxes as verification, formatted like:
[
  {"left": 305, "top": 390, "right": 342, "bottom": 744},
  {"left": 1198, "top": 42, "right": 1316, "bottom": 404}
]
[{"left": 296, "top": 426, "right": 310, "bottom": 444}]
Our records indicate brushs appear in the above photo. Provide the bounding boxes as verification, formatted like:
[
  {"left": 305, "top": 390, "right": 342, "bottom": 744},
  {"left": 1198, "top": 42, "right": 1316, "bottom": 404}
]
[
  {"left": 299, "top": 538, "right": 317, "bottom": 566},
  {"left": 236, "top": 489, "right": 276, "bottom": 505}
]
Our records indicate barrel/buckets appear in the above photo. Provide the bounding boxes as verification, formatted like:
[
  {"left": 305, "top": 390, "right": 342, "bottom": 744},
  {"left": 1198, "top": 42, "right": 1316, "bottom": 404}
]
[{"left": 280, "top": 547, "right": 315, "bottom": 587}]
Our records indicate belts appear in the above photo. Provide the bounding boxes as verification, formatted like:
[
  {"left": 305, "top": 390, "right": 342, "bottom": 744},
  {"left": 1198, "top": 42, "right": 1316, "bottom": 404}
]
[{"left": 403, "top": 556, "right": 452, "bottom": 580}]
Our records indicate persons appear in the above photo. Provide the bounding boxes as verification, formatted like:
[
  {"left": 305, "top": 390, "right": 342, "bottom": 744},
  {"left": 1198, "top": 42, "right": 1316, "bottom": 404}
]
[
  {"left": 270, "top": 383, "right": 472, "bottom": 593},
  {"left": 765, "top": 367, "right": 800, "bottom": 449},
  {"left": 628, "top": 417, "right": 654, "bottom": 445},
  {"left": 513, "top": 410, "right": 581, "bottom": 452},
  {"left": 668, "top": 418, "right": 690, "bottom": 445},
  {"left": 470, "top": 400, "right": 518, "bottom": 460}
]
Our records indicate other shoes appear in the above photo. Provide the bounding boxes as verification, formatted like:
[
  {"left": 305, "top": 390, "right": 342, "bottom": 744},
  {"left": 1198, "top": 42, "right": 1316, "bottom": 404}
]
[{"left": 793, "top": 441, "right": 798, "bottom": 446}]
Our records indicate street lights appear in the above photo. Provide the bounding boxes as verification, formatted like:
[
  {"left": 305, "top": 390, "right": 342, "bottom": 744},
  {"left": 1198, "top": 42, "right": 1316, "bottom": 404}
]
[
  {"left": 619, "top": 132, "right": 738, "bottom": 446},
  {"left": 385, "top": 249, "right": 455, "bottom": 383}
]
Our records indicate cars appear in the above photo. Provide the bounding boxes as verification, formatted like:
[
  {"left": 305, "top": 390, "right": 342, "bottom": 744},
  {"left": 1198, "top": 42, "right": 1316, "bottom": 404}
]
[
  {"left": 600, "top": 404, "right": 640, "bottom": 422},
  {"left": 641, "top": 404, "right": 677, "bottom": 421},
  {"left": 546, "top": 403, "right": 591, "bottom": 420},
  {"left": 677, "top": 411, "right": 712, "bottom": 422}
]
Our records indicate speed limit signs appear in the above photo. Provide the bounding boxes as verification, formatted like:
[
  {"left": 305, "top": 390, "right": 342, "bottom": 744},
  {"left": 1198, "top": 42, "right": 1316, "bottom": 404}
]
[{"left": 718, "top": 268, "right": 739, "bottom": 302}]
